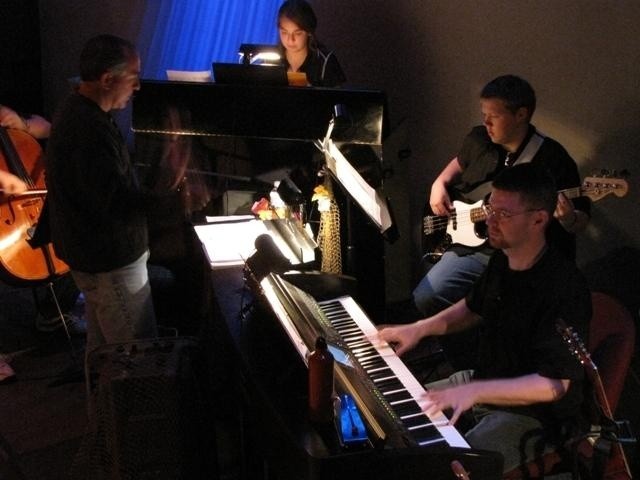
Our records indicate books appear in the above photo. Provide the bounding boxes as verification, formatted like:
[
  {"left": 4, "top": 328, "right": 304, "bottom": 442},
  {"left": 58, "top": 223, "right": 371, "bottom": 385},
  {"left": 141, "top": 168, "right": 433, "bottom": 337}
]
[
  {"left": 166, "top": 69, "right": 211, "bottom": 82},
  {"left": 191, "top": 215, "right": 318, "bottom": 271}
]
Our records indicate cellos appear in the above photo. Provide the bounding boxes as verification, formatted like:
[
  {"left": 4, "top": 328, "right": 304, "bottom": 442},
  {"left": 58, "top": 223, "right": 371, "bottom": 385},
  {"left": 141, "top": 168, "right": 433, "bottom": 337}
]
[{"left": 0, "top": 116, "right": 75, "bottom": 290}]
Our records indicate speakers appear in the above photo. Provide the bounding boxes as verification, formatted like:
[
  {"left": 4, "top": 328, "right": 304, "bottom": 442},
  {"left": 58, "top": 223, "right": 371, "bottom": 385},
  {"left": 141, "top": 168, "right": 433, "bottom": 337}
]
[{"left": 87, "top": 336, "right": 218, "bottom": 479}]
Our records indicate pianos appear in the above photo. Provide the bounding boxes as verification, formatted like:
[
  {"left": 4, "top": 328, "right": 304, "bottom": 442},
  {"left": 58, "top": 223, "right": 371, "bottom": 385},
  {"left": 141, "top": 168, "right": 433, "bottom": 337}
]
[{"left": 243, "top": 266, "right": 505, "bottom": 480}]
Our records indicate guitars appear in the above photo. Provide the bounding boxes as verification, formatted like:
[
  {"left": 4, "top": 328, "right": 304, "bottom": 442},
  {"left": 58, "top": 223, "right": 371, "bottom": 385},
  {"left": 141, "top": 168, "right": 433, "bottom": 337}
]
[
  {"left": 554, "top": 315, "right": 633, "bottom": 479},
  {"left": 420, "top": 167, "right": 629, "bottom": 263}
]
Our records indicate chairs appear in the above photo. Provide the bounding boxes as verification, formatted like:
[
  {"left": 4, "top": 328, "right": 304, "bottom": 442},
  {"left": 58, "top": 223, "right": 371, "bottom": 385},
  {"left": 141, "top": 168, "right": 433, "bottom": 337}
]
[{"left": 506, "top": 290, "right": 636, "bottom": 477}]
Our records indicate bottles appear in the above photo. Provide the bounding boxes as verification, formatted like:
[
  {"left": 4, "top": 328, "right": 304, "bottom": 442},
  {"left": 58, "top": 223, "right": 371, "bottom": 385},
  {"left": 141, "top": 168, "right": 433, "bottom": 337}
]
[{"left": 307, "top": 336, "right": 335, "bottom": 424}]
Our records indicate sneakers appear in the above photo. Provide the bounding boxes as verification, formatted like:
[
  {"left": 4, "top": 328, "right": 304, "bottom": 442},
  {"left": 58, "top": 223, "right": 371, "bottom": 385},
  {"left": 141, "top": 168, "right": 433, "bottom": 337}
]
[
  {"left": 36, "top": 292, "right": 90, "bottom": 334},
  {"left": 1, "top": 360, "right": 14, "bottom": 382}
]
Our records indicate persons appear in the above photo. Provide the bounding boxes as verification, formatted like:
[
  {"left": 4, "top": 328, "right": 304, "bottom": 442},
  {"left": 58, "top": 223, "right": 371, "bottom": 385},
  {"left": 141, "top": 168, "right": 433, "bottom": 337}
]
[
  {"left": 276, "top": 0, "right": 350, "bottom": 89},
  {"left": 362, "top": 162, "right": 592, "bottom": 479},
  {"left": 43, "top": 33, "right": 211, "bottom": 379},
  {"left": 409, "top": 74, "right": 594, "bottom": 369},
  {"left": 1, "top": 104, "right": 86, "bottom": 336}
]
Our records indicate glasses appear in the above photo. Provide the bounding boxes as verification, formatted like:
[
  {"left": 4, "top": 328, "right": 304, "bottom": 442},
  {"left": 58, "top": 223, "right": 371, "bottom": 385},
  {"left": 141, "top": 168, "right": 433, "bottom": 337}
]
[{"left": 482, "top": 205, "right": 539, "bottom": 223}]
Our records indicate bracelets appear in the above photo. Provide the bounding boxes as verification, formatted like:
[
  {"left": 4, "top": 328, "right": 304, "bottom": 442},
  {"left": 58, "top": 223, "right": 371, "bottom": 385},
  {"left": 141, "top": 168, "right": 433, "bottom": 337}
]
[
  {"left": 20, "top": 115, "right": 32, "bottom": 131},
  {"left": 563, "top": 212, "right": 578, "bottom": 228}
]
